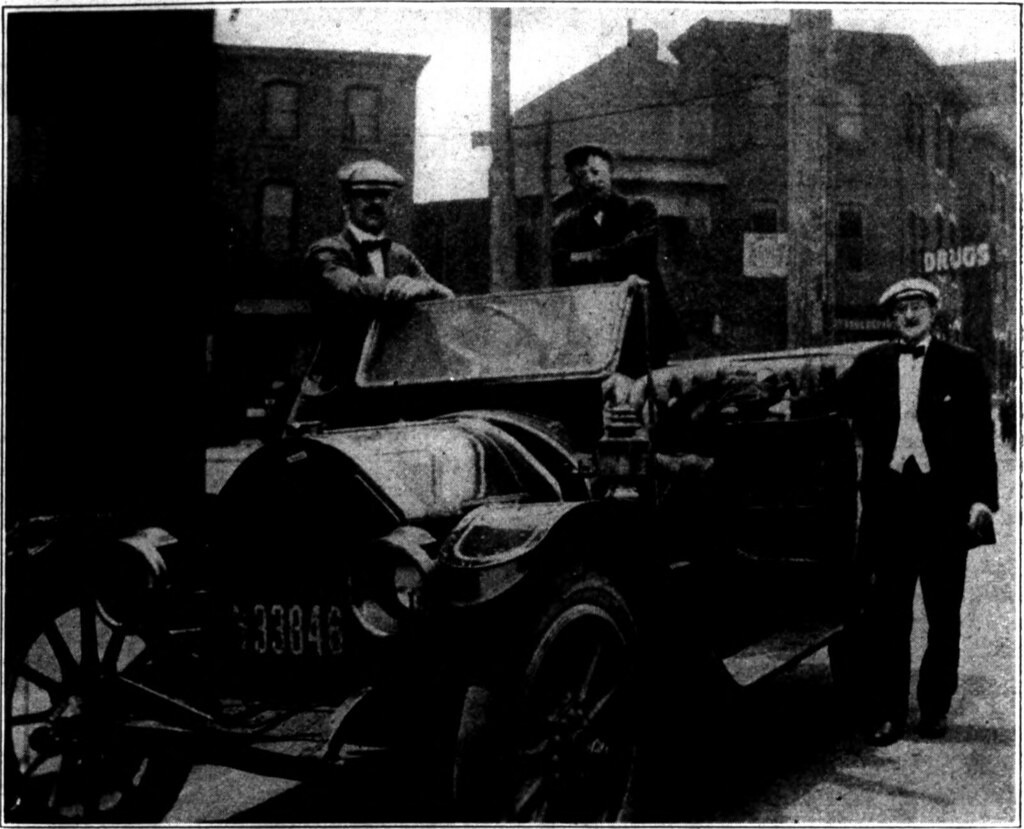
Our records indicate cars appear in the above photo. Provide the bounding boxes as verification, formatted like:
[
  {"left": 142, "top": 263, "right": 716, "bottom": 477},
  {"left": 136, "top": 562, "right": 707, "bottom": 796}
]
[{"left": 0, "top": 277, "right": 997, "bottom": 822}]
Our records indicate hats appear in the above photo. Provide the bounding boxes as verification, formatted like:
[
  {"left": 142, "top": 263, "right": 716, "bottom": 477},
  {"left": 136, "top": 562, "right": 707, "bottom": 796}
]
[
  {"left": 337, "top": 161, "right": 408, "bottom": 190},
  {"left": 880, "top": 279, "right": 942, "bottom": 304},
  {"left": 563, "top": 143, "right": 618, "bottom": 172}
]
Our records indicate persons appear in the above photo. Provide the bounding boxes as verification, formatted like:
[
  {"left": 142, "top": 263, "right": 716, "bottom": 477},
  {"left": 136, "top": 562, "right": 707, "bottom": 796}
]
[
  {"left": 770, "top": 276, "right": 999, "bottom": 748},
  {"left": 548, "top": 144, "right": 676, "bottom": 381},
  {"left": 302, "top": 160, "right": 457, "bottom": 309}
]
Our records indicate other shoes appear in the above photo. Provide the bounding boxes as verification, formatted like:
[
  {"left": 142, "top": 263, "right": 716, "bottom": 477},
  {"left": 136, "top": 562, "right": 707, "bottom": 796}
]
[
  {"left": 917, "top": 714, "right": 949, "bottom": 739},
  {"left": 868, "top": 718, "right": 907, "bottom": 745}
]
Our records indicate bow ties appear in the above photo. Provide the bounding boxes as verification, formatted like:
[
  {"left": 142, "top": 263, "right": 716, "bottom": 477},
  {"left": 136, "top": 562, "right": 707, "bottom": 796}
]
[
  {"left": 360, "top": 238, "right": 393, "bottom": 253},
  {"left": 897, "top": 346, "right": 927, "bottom": 361}
]
